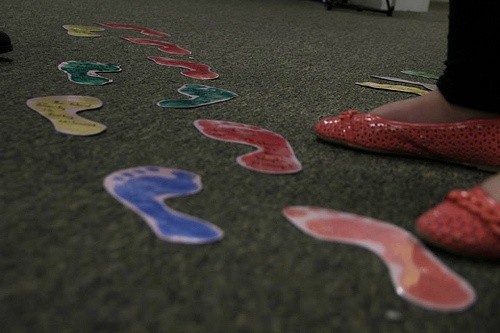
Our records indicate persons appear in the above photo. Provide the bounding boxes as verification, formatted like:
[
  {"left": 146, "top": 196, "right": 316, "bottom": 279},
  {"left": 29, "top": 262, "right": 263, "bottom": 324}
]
[{"left": 314, "top": 0, "right": 499, "bottom": 261}]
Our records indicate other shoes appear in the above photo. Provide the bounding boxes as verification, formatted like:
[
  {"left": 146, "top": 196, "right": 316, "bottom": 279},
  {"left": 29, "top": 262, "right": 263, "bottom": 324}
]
[
  {"left": 413, "top": 185, "right": 500, "bottom": 260},
  {"left": 313, "top": 108, "right": 499, "bottom": 172}
]
